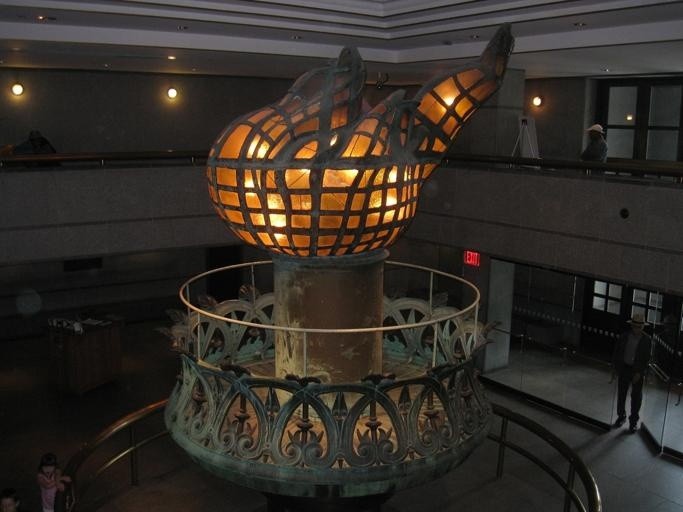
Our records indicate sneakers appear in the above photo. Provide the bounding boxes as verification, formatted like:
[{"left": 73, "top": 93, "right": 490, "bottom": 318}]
[
  {"left": 630, "top": 423, "right": 637, "bottom": 432},
  {"left": 615, "top": 416, "right": 626, "bottom": 428}
]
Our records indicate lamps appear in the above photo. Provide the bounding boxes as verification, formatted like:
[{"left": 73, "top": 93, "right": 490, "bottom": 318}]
[{"left": 205, "top": 22, "right": 518, "bottom": 259}]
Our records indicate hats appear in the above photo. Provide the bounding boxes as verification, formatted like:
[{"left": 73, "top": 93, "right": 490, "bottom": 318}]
[
  {"left": 585, "top": 124, "right": 604, "bottom": 134},
  {"left": 627, "top": 313, "right": 650, "bottom": 326}
]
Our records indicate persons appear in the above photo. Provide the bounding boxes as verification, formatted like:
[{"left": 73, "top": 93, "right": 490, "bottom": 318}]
[
  {"left": 1, "top": 488, "right": 21, "bottom": 512},
  {"left": 15, "top": 130, "right": 58, "bottom": 155},
  {"left": 607, "top": 313, "right": 654, "bottom": 432},
  {"left": 579, "top": 123, "right": 609, "bottom": 164},
  {"left": 37, "top": 453, "right": 71, "bottom": 512}
]
